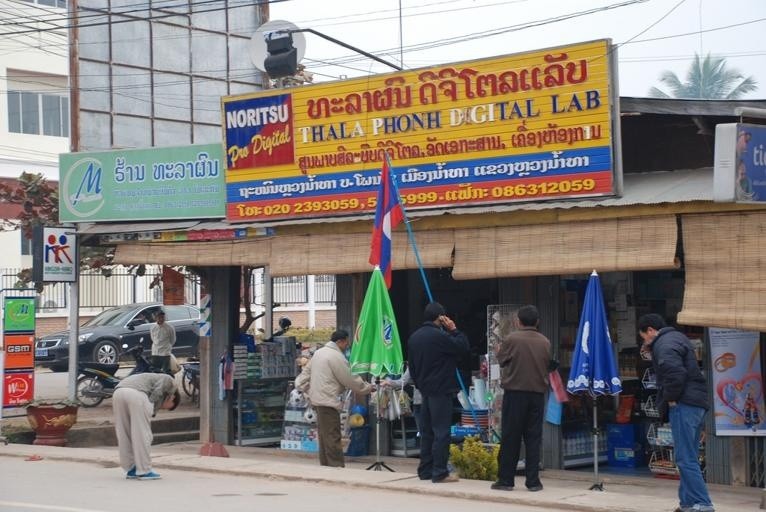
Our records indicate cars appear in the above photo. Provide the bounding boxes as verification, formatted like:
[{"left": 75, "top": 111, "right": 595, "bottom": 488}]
[{"left": 34, "top": 299, "right": 201, "bottom": 376}]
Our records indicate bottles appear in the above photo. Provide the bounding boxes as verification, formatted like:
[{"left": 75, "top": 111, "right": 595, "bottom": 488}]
[
  {"left": 242, "top": 399, "right": 257, "bottom": 423},
  {"left": 620, "top": 367, "right": 637, "bottom": 376},
  {"left": 656, "top": 427, "right": 673, "bottom": 442},
  {"left": 558, "top": 326, "right": 609, "bottom": 456},
  {"left": 743, "top": 393, "right": 760, "bottom": 428}
]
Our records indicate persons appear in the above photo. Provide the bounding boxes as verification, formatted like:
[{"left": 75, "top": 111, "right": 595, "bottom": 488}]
[
  {"left": 489, "top": 304, "right": 555, "bottom": 491},
  {"left": 380, "top": 363, "right": 424, "bottom": 436},
  {"left": 637, "top": 311, "right": 717, "bottom": 512},
  {"left": 112, "top": 372, "right": 180, "bottom": 481},
  {"left": 293, "top": 327, "right": 378, "bottom": 466},
  {"left": 404, "top": 301, "right": 472, "bottom": 483},
  {"left": 137, "top": 311, "right": 150, "bottom": 324},
  {"left": 149, "top": 310, "right": 176, "bottom": 375}
]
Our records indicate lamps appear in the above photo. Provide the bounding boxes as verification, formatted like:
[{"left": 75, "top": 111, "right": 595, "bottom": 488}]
[{"left": 262, "top": 24, "right": 402, "bottom": 81}]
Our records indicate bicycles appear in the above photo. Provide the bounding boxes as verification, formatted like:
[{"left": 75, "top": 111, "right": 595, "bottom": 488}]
[{"left": 178, "top": 349, "right": 202, "bottom": 399}]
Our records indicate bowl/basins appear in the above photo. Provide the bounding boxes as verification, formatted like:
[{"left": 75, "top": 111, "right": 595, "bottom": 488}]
[{"left": 460, "top": 409, "right": 490, "bottom": 429}]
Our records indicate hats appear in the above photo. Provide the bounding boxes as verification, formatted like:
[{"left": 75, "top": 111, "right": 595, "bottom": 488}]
[{"left": 155, "top": 310, "right": 165, "bottom": 318}]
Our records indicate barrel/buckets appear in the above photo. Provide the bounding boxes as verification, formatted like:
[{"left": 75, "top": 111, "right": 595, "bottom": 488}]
[{"left": 616, "top": 394, "right": 636, "bottom": 424}]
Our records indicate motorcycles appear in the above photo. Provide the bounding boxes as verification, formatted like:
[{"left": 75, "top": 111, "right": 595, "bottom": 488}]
[
  {"left": 73, "top": 333, "right": 185, "bottom": 413},
  {"left": 254, "top": 317, "right": 308, "bottom": 351}
]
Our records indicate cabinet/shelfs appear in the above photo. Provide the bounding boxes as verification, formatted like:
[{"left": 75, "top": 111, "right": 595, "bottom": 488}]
[
  {"left": 389, "top": 384, "right": 423, "bottom": 457},
  {"left": 279, "top": 374, "right": 370, "bottom": 458},
  {"left": 225, "top": 369, "right": 294, "bottom": 451},
  {"left": 637, "top": 318, "right": 708, "bottom": 476}
]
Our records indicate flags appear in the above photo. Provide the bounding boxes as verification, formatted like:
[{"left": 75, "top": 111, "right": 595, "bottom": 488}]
[{"left": 368, "top": 155, "right": 406, "bottom": 292}]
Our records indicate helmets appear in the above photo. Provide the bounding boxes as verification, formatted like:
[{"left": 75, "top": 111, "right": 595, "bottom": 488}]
[{"left": 279, "top": 315, "right": 291, "bottom": 329}]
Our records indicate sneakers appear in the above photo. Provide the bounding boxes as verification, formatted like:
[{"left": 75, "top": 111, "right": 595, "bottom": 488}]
[
  {"left": 137, "top": 469, "right": 161, "bottom": 480},
  {"left": 420, "top": 472, "right": 459, "bottom": 483},
  {"left": 126, "top": 464, "right": 137, "bottom": 479},
  {"left": 491, "top": 482, "right": 513, "bottom": 491},
  {"left": 527, "top": 483, "right": 543, "bottom": 491},
  {"left": 674, "top": 506, "right": 715, "bottom": 512}
]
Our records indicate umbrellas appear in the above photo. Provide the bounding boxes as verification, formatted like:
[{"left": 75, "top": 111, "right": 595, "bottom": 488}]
[
  {"left": 567, "top": 268, "right": 624, "bottom": 492},
  {"left": 349, "top": 265, "right": 405, "bottom": 473}
]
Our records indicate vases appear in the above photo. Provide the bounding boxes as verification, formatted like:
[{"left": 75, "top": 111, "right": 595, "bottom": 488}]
[{"left": 26, "top": 404, "right": 79, "bottom": 447}]
